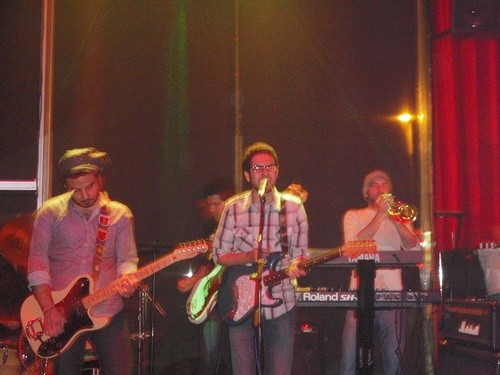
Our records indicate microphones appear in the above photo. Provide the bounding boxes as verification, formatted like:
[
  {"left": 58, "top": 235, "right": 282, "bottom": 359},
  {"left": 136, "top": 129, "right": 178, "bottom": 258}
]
[{"left": 258, "top": 177, "right": 269, "bottom": 198}]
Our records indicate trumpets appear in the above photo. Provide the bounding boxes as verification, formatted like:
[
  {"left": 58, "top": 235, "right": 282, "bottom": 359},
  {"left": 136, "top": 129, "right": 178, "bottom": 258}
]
[{"left": 379, "top": 193, "right": 418, "bottom": 223}]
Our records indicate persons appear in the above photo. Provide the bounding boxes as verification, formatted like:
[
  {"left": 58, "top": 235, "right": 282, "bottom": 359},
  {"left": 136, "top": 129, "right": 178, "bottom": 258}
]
[
  {"left": 341, "top": 168, "right": 421, "bottom": 375},
  {"left": 176, "top": 176, "right": 235, "bottom": 375},
  {"left": 212, "top": 141, "right": 309, "bottom": 375},
  {"left": 27, "top": 147, "right": 140, "bottom": 375}
]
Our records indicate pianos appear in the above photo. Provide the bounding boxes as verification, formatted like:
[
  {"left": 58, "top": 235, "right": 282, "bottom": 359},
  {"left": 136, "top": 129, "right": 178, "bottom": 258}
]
[{"left": 292, "top": 246, "right": 443, "bottom": 375}]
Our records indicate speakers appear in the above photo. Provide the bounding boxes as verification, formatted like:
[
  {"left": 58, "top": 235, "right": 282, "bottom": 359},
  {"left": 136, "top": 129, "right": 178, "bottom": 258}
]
[{"left": 439, "top": 339, "right": 500, "bottom": 375}]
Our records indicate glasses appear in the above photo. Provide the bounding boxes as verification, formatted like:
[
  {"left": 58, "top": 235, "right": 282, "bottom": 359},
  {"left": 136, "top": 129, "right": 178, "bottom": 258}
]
[{"left": 248, "top": 164, "right": 278, "bottom": 173}]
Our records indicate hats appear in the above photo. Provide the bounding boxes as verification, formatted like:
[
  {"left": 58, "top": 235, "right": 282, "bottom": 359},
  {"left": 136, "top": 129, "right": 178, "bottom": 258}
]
[{"left": 362, "top": 169, "right": 393, "bottom": 201}]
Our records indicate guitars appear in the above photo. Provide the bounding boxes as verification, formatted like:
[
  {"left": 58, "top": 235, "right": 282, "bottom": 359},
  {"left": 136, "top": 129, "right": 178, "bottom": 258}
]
[
  {"left": 217, "top": 239, "right": 378, "bottom": 326},
  {"left": 20, "top": 238, "right": 208, "bottom": 359},
  {"left": 187, "top": 183, "right": 308, "bottom": 325}
]
